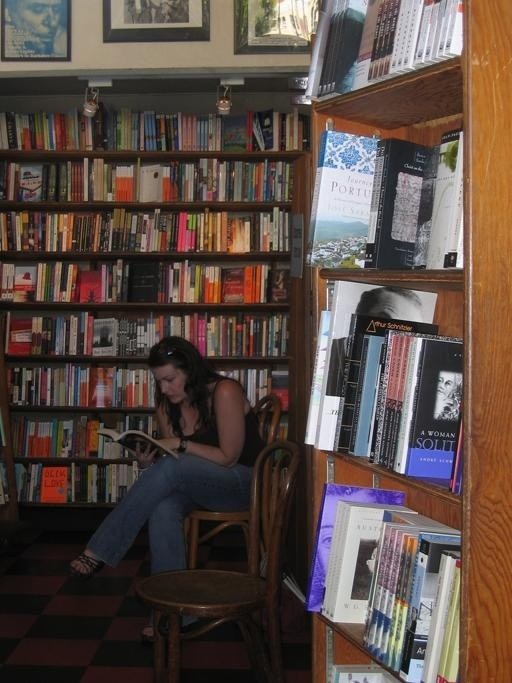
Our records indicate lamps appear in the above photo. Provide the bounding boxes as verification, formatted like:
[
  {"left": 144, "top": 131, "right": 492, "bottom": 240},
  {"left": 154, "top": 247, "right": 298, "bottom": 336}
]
[{"left": 77, "top": 73, "right": 308, "bottom": 118}]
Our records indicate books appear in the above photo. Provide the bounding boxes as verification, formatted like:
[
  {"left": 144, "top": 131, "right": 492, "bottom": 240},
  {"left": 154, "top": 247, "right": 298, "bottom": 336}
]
[
  {"left": 302, "top": 2, "right": 464, "bottom": 274},
  {"left": 304, "top": 279, "right": 461, "bottom": 498},
  {"left": 303, "top": 484, "right": 463, "bottom": 682},
  {"left": 261, "top": 420, "right": 290, "bottom": 444},
  {"left": 1, "top": 107, "right": 302, "bottom": 202},
  {"left": 3, "top": 206, "right": 291, "bottom": 304},
  {"left": 2, "top": 310, "right": 289, "bottom": 411},
  {"left": 2, "top": 414, "right": 181, "bottom": 503}
]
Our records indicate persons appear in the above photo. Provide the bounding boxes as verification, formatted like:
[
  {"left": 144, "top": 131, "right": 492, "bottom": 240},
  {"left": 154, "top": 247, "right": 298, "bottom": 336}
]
[
  {"left": 69, "top": 336, "right": 274, "bottom": 644},
  {"left": 5, "top": 0, "right": 67, "bottom": 58}
]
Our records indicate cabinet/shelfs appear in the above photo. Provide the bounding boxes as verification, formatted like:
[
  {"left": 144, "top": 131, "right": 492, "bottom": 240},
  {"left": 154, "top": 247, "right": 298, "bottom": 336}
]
[
  {"left": 1, "top": 149, "right": 308, "bottom": 509},
  {"left": 307, "top": 0, "right": 511, "bottom": 681}
]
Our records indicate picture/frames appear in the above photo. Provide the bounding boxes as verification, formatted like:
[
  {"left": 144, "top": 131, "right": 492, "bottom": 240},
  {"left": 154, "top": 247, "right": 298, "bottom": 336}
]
[
  {"left": 1, "top": 0, "right": 73, "bottom": 62},
  {"left": 103, "top": 1, "right": 210, "bottom": 43},
  {"left": 233, "top": 1, "right": 320, "bottom": 55}
]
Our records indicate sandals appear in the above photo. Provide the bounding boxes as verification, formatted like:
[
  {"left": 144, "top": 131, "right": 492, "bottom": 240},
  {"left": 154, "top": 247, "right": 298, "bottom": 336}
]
[{"left": 70, "top": 552, "right": 105, "bottom": 576}]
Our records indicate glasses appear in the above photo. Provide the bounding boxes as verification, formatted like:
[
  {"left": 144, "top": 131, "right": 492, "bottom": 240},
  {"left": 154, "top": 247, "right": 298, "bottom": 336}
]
[{"left": 150, "top": 343, "right": 177, "bottom": 355}]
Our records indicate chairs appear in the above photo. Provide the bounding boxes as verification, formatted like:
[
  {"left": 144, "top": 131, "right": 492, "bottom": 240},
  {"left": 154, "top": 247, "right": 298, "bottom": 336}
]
[
  {"left": 128, "top": 439, "right": 300, "bottom": 682},
  {"left": 184, "top": 394, "right": 283, "bottom": 574}
]
